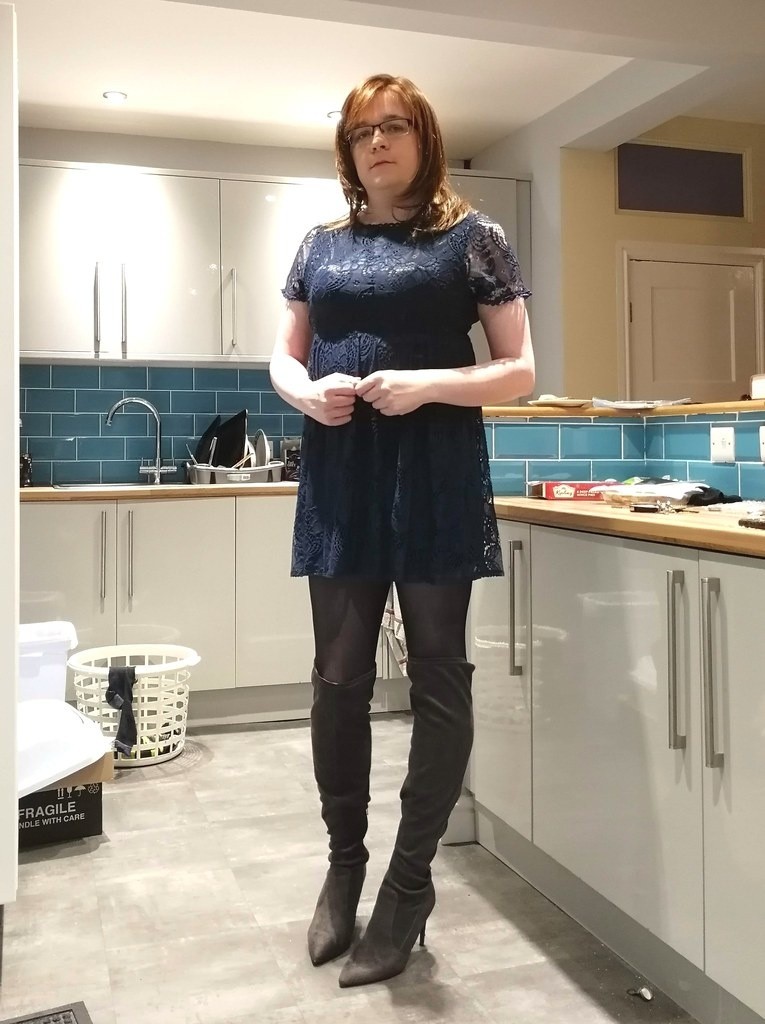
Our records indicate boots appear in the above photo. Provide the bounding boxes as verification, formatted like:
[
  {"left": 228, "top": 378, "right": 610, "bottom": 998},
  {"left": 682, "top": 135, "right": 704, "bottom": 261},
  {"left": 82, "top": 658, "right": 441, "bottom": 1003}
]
[
  {"left": 307, "top": 658, "right": 377, "bottom": 966},
  {"left": 338, "top": 656, "right": 475, "bottom": 988}
]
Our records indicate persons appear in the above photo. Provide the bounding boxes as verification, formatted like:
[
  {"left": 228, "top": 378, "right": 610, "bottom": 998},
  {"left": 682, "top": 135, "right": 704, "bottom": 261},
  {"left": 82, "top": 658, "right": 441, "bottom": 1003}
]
[{"left": 268, "top": 76, "right": 536, "bottom": 989}]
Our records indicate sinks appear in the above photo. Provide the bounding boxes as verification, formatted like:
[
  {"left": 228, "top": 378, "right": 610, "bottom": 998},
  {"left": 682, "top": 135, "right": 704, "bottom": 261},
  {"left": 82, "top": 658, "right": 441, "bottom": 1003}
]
[{"left": 55, "top": 480, "right": 140, "bottom": 488}]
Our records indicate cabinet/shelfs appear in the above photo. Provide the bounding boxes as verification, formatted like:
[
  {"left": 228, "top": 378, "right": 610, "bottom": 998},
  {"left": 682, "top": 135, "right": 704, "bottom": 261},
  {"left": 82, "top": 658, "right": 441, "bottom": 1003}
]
[
  {"left": 19, "top": 500, "right": 413, "bottom": 729},
  {"left": 439, "top": 517, "right": 765, "bottom": 1024},
  {"left": 19, "top": 156, "right": 534, "bottom": 371}
]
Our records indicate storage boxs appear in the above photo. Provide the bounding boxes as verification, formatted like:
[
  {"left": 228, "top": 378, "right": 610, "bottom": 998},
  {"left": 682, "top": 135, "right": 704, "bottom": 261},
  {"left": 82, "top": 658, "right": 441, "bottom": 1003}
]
[{"left": 18, "top": 748, "right": 115, "bottom": 851}]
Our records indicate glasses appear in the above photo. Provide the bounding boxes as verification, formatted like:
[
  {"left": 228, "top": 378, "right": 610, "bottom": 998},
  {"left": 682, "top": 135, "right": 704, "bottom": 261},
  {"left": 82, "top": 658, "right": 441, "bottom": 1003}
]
[{"left": 345, "top": 118, "right": 419, "bottom": 146}]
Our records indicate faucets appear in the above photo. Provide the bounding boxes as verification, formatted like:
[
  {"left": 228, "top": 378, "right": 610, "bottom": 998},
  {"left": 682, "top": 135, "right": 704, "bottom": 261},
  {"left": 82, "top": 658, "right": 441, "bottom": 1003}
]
[{"left": 105, "top": 395, "right": 177, "bottom": 485}]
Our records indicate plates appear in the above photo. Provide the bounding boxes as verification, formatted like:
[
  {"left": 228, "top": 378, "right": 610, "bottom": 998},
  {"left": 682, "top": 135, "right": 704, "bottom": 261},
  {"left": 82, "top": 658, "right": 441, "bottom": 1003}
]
[
  {"left": 192, "top": 408, "right": 270, "bottom": 467},
  {"left": 527, "top": 399, "right": 594, "bottom": 407}
]
